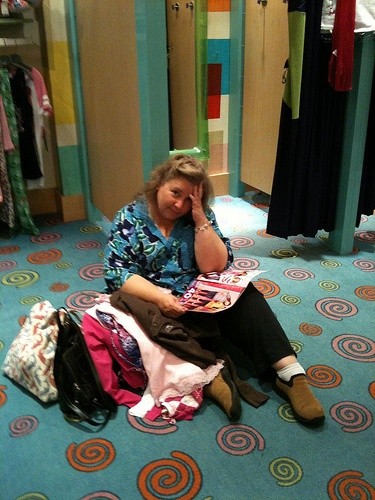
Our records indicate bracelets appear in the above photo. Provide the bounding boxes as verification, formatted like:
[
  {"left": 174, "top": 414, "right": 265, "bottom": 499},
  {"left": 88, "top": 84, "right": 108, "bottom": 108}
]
[{"left": 194, "top": 221, "right": 213, "bottom": 233}]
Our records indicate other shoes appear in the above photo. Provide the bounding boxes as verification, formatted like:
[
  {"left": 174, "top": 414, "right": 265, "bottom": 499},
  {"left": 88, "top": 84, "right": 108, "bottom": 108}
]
[
  {"left": 274, "top": 372, "right": 326, "bottom": 425},
  {"left": 203, "top": 364, "right": 241, "bottom": 420}
]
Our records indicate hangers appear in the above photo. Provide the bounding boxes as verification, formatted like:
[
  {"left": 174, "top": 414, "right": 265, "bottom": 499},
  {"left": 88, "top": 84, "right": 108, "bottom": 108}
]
[{"left": 0, "top": 37, "right": 33, "bottom": 81}]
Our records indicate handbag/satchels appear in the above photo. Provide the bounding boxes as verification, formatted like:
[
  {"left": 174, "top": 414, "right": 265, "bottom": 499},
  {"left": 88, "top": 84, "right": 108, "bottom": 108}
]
[{"left": 2, "top": 299, "right": 118, "bottom": 426}]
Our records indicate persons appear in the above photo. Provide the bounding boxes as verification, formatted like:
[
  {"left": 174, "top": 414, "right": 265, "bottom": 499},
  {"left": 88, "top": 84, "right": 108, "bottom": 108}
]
[
  {"left": 103, "top": 153, "right": 325, "bottom": 425},
  {"left": 182, "top": 272, "right": 247, "bottom": 312}
]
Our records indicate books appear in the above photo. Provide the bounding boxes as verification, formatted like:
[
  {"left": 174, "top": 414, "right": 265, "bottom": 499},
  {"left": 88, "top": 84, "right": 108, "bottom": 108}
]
[{"left": 179, "top": 270, "right": 267, "bottom": 314}]
